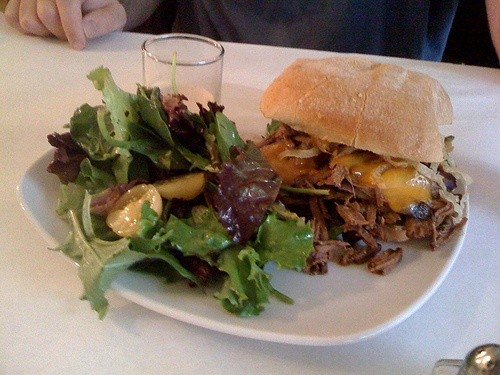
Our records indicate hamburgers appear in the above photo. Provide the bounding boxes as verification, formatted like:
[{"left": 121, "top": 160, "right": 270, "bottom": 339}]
[{"left": 258, "top": 55, "right": 471, "bottom": 250}]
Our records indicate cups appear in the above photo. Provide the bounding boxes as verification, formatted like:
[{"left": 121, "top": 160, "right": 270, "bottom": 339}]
[{"left": 142, "top": 32, "right": 224, "bottom": 110}]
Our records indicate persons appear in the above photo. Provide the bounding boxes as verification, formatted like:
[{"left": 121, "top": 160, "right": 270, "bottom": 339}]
[{"left": 1, "top": 0, "right": 500, "bottom": 63}]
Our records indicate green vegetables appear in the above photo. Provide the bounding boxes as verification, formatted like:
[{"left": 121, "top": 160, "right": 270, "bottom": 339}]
[{"left": 46, "top": 65, "right": 331, "bottom": 322}]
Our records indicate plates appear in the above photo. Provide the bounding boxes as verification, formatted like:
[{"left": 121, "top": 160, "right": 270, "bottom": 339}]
[{"left": 18, "top": 81, "right": 469, "bottom": 347}]
